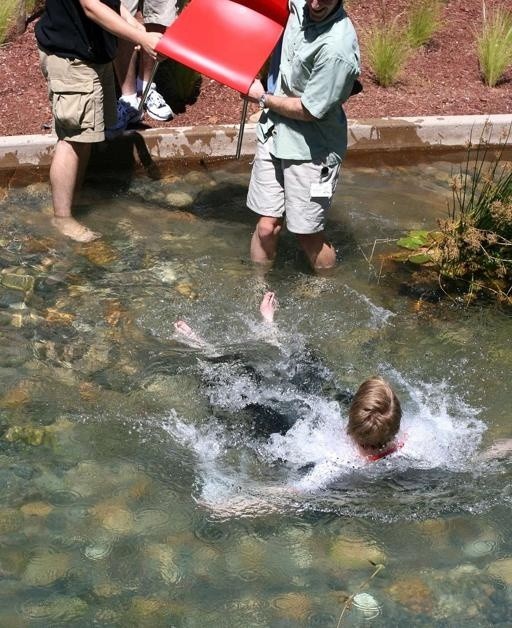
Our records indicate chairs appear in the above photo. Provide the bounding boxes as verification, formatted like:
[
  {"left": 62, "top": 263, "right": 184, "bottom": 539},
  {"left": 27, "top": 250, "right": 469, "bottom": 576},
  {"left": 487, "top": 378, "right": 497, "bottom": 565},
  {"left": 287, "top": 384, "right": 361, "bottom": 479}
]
[{"left": 135, "top": 0, "right": 295, "bottom": 164}]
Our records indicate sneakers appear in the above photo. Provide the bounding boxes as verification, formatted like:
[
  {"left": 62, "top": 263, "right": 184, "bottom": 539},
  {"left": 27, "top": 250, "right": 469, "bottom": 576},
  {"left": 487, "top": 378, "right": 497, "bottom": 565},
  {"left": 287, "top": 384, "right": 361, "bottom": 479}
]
[
  {"left": 105, "top": 96, "right": 144, "bottom": 135},
  {"left": 136, "top": 78, "right": 174, "bottom": 121}
]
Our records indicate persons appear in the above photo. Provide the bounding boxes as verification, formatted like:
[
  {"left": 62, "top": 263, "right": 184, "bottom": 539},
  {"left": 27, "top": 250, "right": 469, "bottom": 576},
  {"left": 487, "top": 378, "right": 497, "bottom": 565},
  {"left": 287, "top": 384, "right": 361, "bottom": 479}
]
[
  {"left": 34, "top": 1, "right": 167, "bottom": 245},
  {"left": 112, "top": 1, "right": 179, "bottom": 125},
  {"left": 173, "top": 292, "right": 512, "bottom": 498},
  {"left": 239, "top": 1, "right": 362, "bottom": 273}
]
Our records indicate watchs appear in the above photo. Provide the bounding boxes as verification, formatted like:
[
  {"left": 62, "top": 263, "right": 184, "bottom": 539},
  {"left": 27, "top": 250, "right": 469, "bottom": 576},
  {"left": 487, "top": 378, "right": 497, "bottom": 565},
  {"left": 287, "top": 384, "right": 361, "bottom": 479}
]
[{"left": 258, "top": 90, "right": 273, "bottom": 109}]
